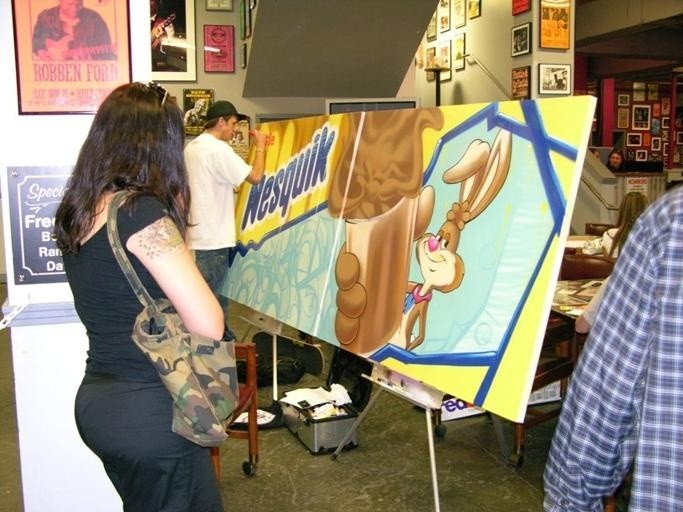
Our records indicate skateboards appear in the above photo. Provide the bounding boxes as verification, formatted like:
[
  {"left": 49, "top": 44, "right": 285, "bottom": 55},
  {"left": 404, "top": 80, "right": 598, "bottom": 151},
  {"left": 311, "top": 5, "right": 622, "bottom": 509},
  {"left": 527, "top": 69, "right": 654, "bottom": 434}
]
[{"left": 253, "top": 331, "right": 324, "bottom": 376}]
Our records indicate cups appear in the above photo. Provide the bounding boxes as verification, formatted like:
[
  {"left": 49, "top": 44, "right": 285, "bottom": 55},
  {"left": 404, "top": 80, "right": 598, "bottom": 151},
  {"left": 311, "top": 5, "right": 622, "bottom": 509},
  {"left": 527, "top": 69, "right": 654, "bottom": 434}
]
[{"left": 339, "top": 192, "right": 423, "bottom": 353}]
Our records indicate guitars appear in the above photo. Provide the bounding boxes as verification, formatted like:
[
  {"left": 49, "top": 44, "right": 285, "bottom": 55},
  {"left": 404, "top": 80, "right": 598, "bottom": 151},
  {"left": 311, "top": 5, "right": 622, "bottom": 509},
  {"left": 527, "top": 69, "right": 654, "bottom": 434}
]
[
  {"left": 151, "top": 13, "right": 176, "bottom": 48},
  {"left": 46, "top": 37, "right": 115, "bottom": 61}
]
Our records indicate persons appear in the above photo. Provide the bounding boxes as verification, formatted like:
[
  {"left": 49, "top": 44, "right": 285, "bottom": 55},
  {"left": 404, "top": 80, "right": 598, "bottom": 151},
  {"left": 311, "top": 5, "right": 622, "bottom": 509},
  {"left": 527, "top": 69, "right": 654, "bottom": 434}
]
[
  {"left": 178, "top": 101, "right": 267, "bottom": 342},
  {"left": 540, "top": 181, "right": 682, "bottom": 509},
  {"left": 603, "top": 191, "right": 647, "bottom": 259},
  {"left": 607, "top": 150, "right": 626, "bottom": 172},
  {"left": 590, "top": 148, "right": 601, "bottom": 160},
  {"left": 53, "top": 80, "right": 231, "bottom": 511},
  {"left": 574, "top": 276, "right": 610, "bottom": 334},
  {"left": 184, "top": 99, "right": 207, "bottom": 127},
  {"left": 32, "top": 0, "right": 116, "bottom": 60}
]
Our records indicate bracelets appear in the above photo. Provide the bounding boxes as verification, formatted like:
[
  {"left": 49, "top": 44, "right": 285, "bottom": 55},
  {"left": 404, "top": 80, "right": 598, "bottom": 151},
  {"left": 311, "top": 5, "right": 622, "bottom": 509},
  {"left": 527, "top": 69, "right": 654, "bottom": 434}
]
[{"left": 256, "top": 147, "right": 267, "bottom": 156}]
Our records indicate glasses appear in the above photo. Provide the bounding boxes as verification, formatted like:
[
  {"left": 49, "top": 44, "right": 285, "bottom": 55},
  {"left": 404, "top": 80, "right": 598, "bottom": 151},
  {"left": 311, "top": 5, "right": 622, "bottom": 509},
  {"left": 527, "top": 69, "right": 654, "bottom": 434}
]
[{"left": 147, "top": 78, "right": 170, "bottom": 107}]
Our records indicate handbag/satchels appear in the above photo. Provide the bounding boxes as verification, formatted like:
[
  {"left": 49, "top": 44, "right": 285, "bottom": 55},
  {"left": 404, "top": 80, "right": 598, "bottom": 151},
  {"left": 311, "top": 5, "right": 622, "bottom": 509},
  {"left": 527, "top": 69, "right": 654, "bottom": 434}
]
[
  {"left": 128, "top": 297, "right": 240, "bottom": 449},
  {"left": 237, "top": 353, "right": 305, "bottom": 388}
]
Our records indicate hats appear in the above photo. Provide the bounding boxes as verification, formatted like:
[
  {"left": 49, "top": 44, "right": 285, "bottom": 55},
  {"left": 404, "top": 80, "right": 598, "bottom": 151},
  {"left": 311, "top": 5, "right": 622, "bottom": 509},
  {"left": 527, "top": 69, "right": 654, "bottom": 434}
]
[{"left": 206, "top": 100, "right": 248, "bottom": 121}]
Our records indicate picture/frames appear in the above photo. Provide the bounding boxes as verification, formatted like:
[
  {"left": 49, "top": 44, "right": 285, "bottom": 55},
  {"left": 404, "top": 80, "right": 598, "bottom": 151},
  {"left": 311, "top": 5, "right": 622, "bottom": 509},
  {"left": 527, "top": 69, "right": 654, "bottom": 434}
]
[
  {"left": 416, "top": 0, "right": 572, "bottom": 99},
  {"left": 617, "top": 78, "right": 683, "bottom": 163},
  {"left": 145, "top": 0, "right": 257, "bottom": 83}
]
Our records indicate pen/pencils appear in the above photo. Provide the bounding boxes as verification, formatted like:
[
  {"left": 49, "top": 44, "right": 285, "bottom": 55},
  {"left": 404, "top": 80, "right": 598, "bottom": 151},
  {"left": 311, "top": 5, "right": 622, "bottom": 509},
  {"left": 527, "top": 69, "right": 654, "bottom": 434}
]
[
  {"left": 377, "top": 378, "right": 406, "bottom": 394},
  {"left": 248, "top": 130, "right": 255, "bottom": 136}
]
